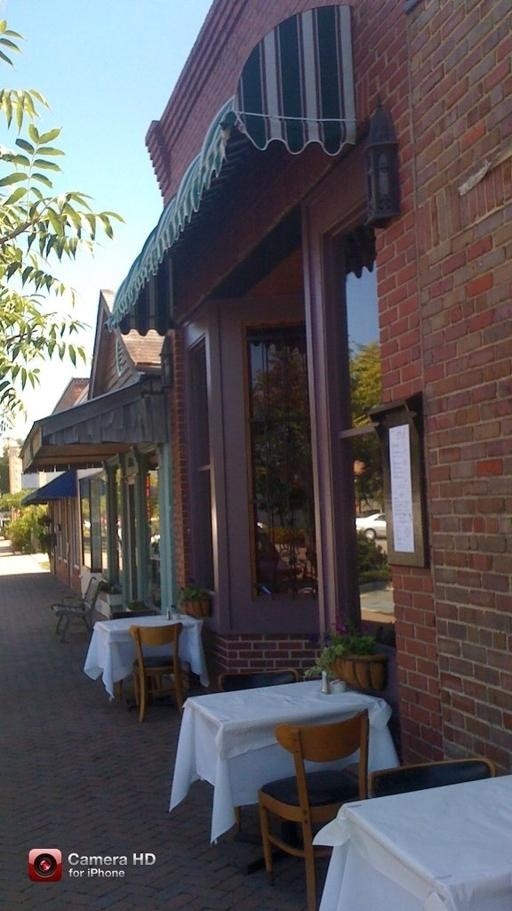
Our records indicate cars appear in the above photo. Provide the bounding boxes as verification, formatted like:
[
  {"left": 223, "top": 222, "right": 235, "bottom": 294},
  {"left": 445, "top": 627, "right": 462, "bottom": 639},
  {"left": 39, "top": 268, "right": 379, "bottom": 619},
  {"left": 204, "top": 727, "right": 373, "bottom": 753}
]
[{"left": 355, "top": 510, "right": 391, "bottom": 542}]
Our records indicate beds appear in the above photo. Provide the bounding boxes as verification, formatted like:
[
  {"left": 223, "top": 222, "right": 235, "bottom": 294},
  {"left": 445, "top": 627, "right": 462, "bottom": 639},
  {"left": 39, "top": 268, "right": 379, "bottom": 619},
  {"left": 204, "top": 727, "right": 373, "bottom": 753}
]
[{"left": 83, "top": 612, "right": 211, "bottom": 704}]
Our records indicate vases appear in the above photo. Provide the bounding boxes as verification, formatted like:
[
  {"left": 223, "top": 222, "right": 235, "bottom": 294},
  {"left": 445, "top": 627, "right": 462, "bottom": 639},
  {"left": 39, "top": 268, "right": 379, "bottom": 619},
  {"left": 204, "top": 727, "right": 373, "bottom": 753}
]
[{"left": 335, "top": 651, "right": 388, "bottom": 691}]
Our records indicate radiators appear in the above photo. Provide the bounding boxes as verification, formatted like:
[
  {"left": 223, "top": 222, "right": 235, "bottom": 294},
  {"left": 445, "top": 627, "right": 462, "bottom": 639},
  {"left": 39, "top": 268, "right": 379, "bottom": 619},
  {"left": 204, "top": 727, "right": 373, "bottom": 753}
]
[{"left": 301, "top": 618, "right": 382, "bottom": 680}]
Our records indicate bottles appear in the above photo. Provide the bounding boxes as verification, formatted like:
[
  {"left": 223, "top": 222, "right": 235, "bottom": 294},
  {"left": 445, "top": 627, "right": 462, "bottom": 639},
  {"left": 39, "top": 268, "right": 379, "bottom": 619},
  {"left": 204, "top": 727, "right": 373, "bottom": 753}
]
[
  {"left": 166, "top": 607, "right": 172, "bottom": 620},
  {"left": 320, "top": 670, "right": 329, "bottom": 694}
]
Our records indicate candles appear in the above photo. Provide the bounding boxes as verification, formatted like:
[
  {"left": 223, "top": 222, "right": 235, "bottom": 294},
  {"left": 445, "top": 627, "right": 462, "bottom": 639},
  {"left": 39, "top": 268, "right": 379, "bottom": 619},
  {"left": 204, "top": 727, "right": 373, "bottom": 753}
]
[
  {"left": 166, "top": 607, "right": 170, "bottom": 620},
  {"left": 321, "top": 670, "right": 330, "bottom": 694}
]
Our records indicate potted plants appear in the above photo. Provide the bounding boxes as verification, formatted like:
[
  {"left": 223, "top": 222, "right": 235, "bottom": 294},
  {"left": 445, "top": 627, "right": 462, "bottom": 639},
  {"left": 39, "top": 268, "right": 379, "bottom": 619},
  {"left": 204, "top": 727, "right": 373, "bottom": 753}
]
[{"left": 176, "top": 585, "right": 212, "bottom": 618}]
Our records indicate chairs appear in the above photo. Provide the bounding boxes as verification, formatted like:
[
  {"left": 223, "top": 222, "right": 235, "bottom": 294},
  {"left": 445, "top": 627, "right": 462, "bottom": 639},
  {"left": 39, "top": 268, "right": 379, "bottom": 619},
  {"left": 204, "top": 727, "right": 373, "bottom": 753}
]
[
  {"left": 114, "top": 610, "right": 164, "bottom": 701},
  {"left": 51, "top": 577, "right": 102, "bottom": 642},
  {"left": 258, "top": 708, "right": 369, "bottom": 911},
  {"left": 129, "top": 622, "right": 184, "bottom": 723},
  {"left": 367, "top": 757, "right": 496, "bottom": 799},
  {"left": 217, "top": 669, "right": 300, "bottom": 832}
]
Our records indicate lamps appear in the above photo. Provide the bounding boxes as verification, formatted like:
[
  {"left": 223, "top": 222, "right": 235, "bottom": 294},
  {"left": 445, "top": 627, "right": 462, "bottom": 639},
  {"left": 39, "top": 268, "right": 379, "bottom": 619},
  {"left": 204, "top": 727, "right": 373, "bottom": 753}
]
[
  {"left": 364, "top": 94, "right": 402, "bottom": 229},
  {"left": 159, "top": 336, "right": 174, "bottom": 389}
]
